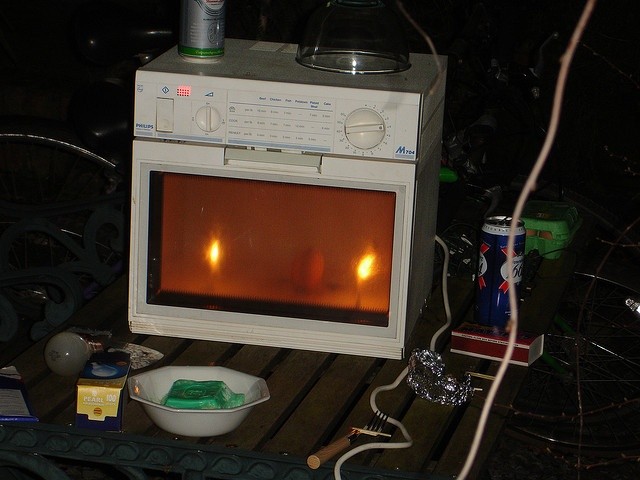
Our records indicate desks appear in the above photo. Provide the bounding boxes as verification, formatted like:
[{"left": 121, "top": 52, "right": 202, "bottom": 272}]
[{"left": 1, "top": 194, "right": 610, "bottom": 480}]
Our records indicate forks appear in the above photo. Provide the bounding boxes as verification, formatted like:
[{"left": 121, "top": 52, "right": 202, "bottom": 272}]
[{"left": 306, "top": 410, "right": 389, "bottom": 469}]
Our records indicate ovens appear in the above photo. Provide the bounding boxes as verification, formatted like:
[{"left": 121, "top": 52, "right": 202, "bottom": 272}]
[{"left": 129, "top": 37, "right": 449, "bottom": 361}]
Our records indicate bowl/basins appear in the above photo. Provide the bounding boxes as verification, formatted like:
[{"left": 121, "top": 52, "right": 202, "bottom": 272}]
[
  {"left": 295, "top": 0, "right": 412, "bottom": 73},
  {"left": 127, "top": 366, "right": 271, "bottom": 437}
]
